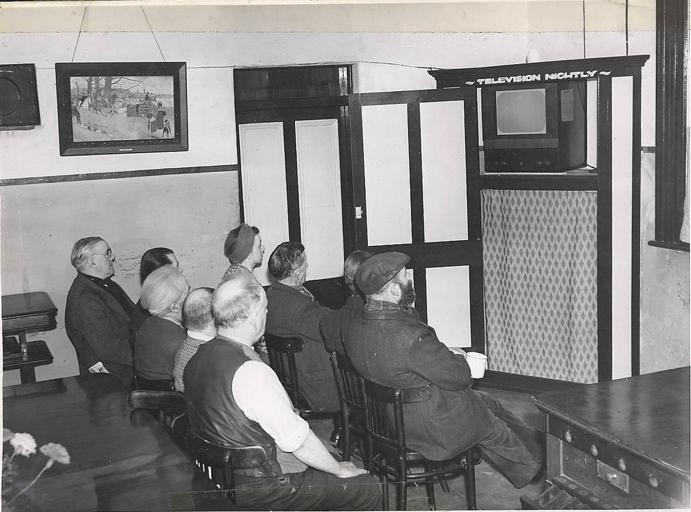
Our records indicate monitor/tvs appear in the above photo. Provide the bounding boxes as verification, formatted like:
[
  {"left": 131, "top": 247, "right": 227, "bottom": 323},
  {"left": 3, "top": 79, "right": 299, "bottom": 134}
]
[{"left": 481, "top": 79, "right": 587, "bottom": 173}]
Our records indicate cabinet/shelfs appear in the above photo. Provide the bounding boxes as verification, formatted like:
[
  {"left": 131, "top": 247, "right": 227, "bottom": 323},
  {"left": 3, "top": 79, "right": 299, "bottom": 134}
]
[{"left": 2, "top": 291, "right": 59, "bottom": 384}]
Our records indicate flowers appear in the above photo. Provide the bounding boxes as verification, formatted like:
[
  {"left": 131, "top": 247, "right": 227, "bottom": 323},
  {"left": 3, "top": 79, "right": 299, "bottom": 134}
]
[{"left": 2, "top": 427, "right": 72, "bottom": 510}]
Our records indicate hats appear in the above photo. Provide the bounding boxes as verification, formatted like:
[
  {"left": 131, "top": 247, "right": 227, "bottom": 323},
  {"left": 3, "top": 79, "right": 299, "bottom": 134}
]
[{"left": 356, "top": 252, "right": 411, "bottom": 295}]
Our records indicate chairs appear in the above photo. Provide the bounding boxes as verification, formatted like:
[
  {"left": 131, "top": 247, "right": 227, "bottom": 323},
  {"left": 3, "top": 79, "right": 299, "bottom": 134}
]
[
  {"left": 181, "top": 422, "right": 267, "bottom": 492},
  {"left": 326, "top": 348, "right": 481, "bottom": 510},
  {"left": 253, "top": 332, "right": 344, "bottom": 448},
  {"left": 131, "top": 391, "right": 184, "bottom": 439}
]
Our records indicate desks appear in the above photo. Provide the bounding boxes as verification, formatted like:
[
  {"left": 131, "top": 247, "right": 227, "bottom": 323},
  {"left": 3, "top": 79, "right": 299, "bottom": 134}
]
[
  {"left": 519, "top": 365, "right": 690, "bottom": 509},
  {"left": 3, "top": 372, "right": 238, "bottom": 512}
]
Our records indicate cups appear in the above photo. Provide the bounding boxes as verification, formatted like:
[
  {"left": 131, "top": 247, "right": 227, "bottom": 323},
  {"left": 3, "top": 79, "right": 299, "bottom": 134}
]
[{"left": 467, "top": 351, "right": 489, "bottom": 380}]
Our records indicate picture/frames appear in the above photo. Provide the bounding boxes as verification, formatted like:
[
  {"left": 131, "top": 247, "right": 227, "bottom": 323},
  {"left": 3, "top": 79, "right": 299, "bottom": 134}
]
[{"left": 54, "top": 61, "right": 189, "bottom": 157}]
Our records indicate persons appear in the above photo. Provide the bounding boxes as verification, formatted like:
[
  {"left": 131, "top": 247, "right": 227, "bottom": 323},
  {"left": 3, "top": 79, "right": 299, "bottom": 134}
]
[
  {"left": 72, "top": 90, "right": 171, "bottom": 139},
  {"left": 133, "top": 248, "right": 183, "bottom": 315},
  {"left": 265, "top": 241, "right": 337, "bottom": 411},
  {"left": 212, "top": 218, "right": 269, "bottom": 350},
  {"left": 339, "top": 251, "right": 547, "bottom": 490},
  {"left": 170, "top": 287, "right": 217, "bottom": 395},
  {"left": 318, "top": 247, "right": 372, "bottom": 360},
  {"left": 133, "top": 263, "right": 189, "bottom": 379},
  {"left": 181, "top": 276, "right": 384, "bottom": 512},
  {"left": 63, "top": 236, "right": 146, "bottom": 393}
]
